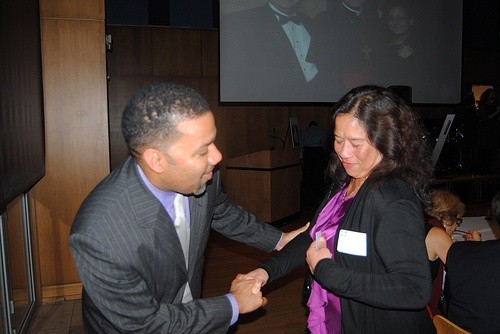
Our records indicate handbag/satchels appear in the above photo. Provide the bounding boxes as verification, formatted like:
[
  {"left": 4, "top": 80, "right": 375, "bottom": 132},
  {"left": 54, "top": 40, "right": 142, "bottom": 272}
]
[{"left": 439, "top": 293, "right": 448, "bottom": 316}]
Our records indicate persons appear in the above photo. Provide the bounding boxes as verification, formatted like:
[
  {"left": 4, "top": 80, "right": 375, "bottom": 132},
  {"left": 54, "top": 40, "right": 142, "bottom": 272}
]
[
  {"left": 423, "top": 189, "right": 500, "bottom": 334},
  {"left": 237, "top": 85, "right": 436, "bottom": 334},
  {"left": 69, "top": 78, "right": 311, "bottom": 334},
  {"left": 219, "top": 0, "right": 451, "bottom": 103}
]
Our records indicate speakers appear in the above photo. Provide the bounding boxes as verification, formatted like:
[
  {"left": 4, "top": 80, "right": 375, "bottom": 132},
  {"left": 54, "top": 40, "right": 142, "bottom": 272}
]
[{"left": 421, "top": 117, "right": 465, "bottom": 179}]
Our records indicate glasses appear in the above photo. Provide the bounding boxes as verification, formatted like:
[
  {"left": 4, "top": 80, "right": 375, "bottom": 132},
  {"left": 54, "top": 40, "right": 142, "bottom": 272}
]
[{"left": 454, "top": 216, "right": 463, "bottom": 225}]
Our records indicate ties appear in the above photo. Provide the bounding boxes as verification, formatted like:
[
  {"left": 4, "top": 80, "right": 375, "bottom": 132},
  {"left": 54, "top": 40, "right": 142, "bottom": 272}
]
[{"left": 173, "top": 194, "right": 194, "bottom": 304}]
